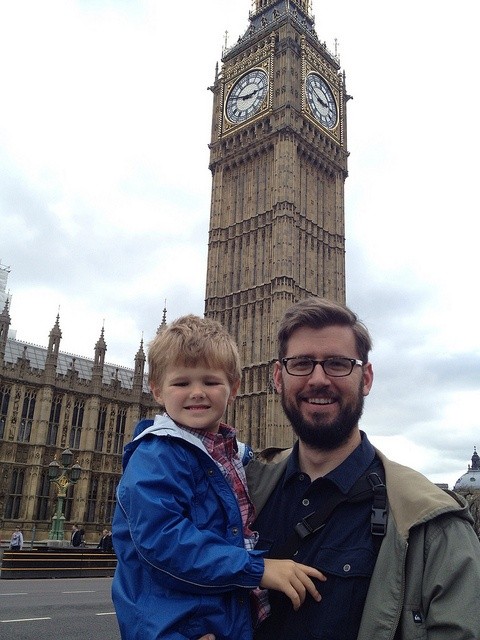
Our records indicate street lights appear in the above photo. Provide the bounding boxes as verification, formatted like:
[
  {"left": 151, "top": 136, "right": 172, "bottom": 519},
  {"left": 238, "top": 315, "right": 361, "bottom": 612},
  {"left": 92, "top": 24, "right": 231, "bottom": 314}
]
[{"left": 48, "top": 447, "right": 82, "bottom": 539}]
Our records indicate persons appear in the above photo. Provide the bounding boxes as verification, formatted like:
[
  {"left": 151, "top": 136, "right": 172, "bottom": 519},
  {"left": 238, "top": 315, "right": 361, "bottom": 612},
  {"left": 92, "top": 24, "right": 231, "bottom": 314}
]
[
  {"left": 109, "top": 313, "right": 328, "bottom": 640},
  {"left": 97, "top": 528, "right": 113, "bottom": 551},
  {"left": 243, "top": 296, "right": 479, "bottom": 639},
  {"left": 108, "top": 530, "right": 113, "bottom": 551},
  {"left": 78, "top": 525, "right": 86, "bottom": 539},
  {"left": 69, "top": 522, "right": 82, "bottom": 547},
  {"left": 9, "top": 524, "right": 24, "bottom": 549}
]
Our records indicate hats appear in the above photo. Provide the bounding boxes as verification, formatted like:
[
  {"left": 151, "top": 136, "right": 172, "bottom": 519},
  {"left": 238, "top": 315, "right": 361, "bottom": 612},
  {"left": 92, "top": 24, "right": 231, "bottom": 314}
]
[{"left": 16, "top": 525, "right": 19, "bottom": 528}]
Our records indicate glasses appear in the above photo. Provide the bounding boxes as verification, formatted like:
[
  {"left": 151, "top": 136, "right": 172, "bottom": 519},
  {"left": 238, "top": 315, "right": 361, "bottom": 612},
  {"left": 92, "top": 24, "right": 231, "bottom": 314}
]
[{"left": 279, "top": 356, "right": 365, "bottom": 378}]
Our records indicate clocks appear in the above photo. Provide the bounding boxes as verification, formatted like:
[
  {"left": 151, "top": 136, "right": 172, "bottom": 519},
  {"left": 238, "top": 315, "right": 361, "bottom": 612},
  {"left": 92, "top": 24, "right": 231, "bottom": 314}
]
[
  {"left": 304, "top": 70, "right": 340, "bottom": 132},
  {"left": 223, "top": 66, "right": 270, "bottom": 125}
]
